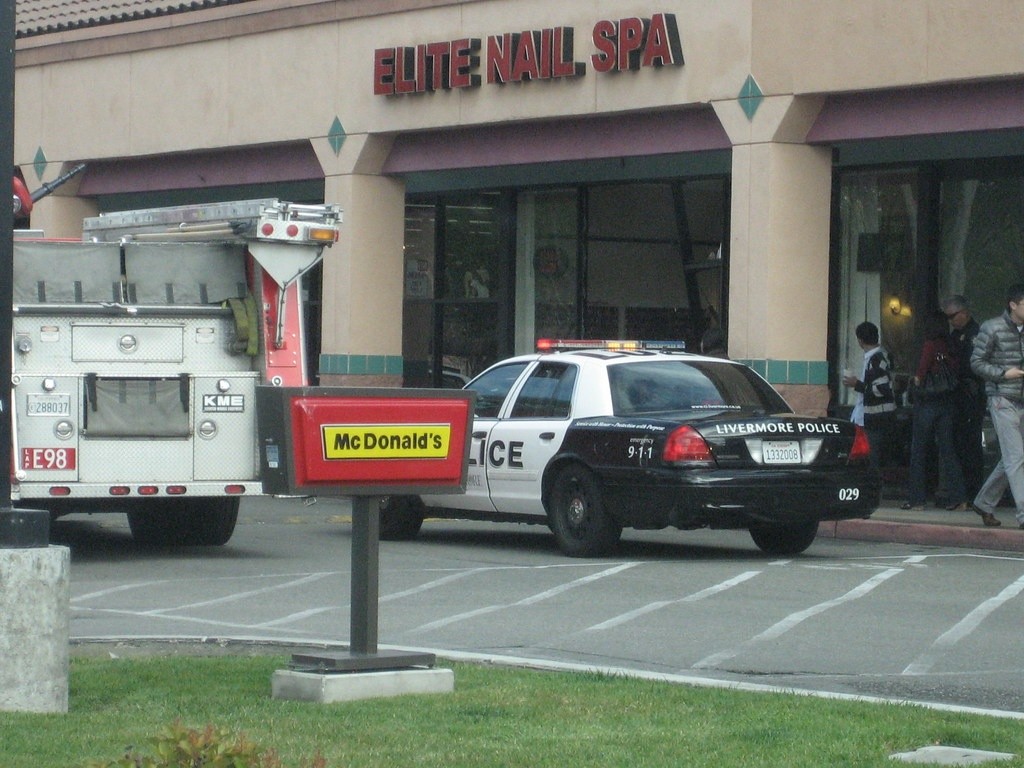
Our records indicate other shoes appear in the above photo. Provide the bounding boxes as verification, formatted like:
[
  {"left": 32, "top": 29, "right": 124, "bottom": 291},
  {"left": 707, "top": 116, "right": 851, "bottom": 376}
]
[
  {"left": 972, "top": 504, "right": 1001, "bottom": 526},
  {"left": 900, "top": 500, "right": 927, "bottom": 510},
  {"left": 946, "top": 496, "right": 967, "bottom": 509}
]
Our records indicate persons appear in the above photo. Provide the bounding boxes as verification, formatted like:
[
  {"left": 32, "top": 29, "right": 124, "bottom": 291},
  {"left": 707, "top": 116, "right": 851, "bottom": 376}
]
[
  {"left": 843, "top": 322, "right": 898, "bottom": 503},
  {"left": 970, "top": 285, "right": 1024, "bottom": 529},
  {"left": 701, "top": 328, "right": 731, "bottom": 359},
  {"left": 899, "top": 293, "right": 985, "bottom": 512}
]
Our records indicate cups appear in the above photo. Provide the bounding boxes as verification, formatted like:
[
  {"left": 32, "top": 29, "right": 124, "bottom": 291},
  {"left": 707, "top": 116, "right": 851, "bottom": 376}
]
[{"left": 843, "top": 369, "right": 853, "bottom": 381}]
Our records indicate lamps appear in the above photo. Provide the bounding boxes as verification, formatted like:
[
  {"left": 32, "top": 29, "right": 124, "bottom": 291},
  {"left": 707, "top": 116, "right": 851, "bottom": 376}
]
[{"left": 888, "top": 293, "right": 903, "bottom": 314}]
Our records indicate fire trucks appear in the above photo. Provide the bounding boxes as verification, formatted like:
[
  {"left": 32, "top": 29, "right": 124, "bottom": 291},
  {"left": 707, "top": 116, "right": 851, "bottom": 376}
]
[{"left": 12, "top": 161, "right": 341, "bottom": 547}]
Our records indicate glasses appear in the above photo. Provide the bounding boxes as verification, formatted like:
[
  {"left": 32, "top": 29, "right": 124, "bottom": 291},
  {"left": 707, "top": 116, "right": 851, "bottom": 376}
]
[{"left": 948, "top": 308, "right": 965, "bottom": 320}]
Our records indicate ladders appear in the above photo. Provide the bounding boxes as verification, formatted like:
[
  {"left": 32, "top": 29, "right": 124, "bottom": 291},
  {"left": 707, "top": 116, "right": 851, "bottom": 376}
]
[{"left": 83, "top": 198, "right": 342, "bottom": 240}]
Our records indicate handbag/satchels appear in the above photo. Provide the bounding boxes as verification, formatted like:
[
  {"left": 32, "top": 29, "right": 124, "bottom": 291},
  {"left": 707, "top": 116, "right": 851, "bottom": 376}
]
[
  {"left": 915, "top": 341, "right": 965, "bottom": 398},
  {"left": 850, "top": 405, "right": 864, "bottom": 426}
]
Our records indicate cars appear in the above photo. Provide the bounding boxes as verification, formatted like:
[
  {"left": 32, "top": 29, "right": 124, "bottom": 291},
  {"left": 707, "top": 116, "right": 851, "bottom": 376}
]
[
  {"left": 376, "top": 339, "right": 884, "bottom": 558},
  {"left": 428, "top": 365, "right": 470, "bottom": 391}
]
